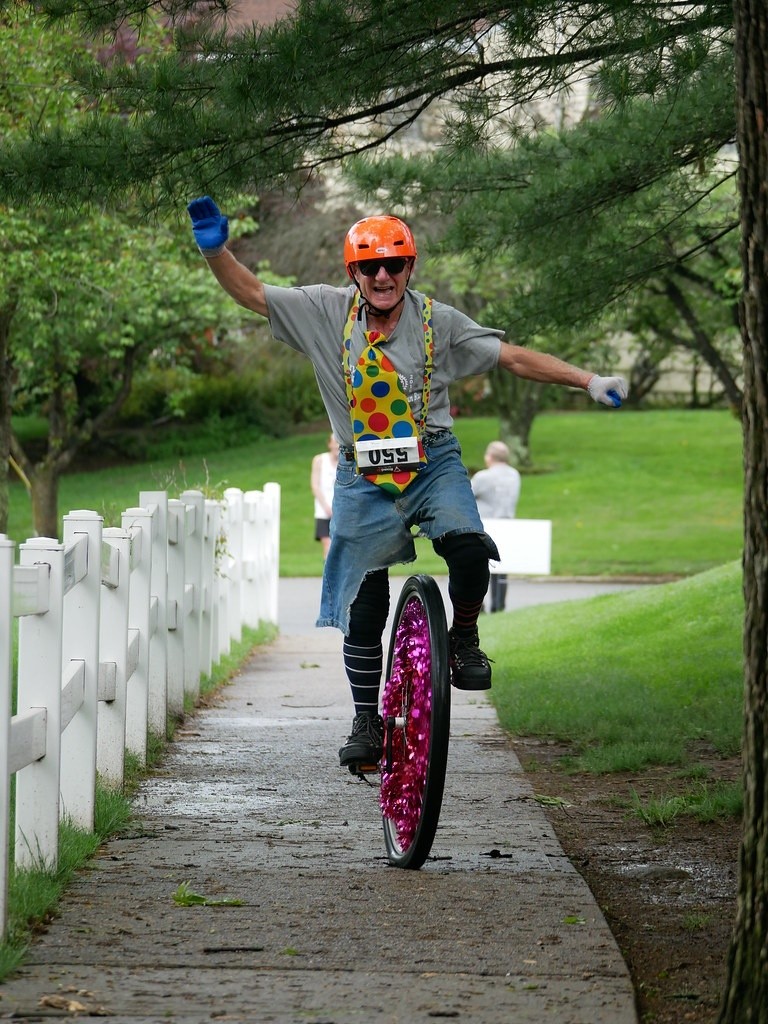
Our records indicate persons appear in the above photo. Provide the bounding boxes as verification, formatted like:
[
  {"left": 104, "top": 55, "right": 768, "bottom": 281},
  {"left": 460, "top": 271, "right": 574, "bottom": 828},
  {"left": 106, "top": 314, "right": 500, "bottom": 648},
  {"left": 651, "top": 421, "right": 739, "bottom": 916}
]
[
  {"left": 186, "top": 194, "right": 629, "bottom": 766},
  {"left": 311, "top": 432, "right": 342, "bottom": 564},
  {"left": 468, "top": 440, "right": 521, "bottom": 614}
]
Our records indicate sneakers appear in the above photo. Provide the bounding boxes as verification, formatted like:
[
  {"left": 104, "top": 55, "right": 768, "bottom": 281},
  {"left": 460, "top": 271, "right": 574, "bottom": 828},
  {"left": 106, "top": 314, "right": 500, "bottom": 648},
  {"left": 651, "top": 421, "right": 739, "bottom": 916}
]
[
  {"left": 448, "top": 627, "right": 492, "bottom": 690},
  {"left": 340, "top": 712, "right": 385, "bottom": 766}
]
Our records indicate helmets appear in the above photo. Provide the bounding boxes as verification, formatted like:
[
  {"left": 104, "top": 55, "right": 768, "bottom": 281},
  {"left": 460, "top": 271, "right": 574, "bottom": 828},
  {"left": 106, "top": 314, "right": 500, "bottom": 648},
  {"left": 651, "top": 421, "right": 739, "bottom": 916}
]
[{"left": 344, "top": 215, "right": 418, "bottom": 280}]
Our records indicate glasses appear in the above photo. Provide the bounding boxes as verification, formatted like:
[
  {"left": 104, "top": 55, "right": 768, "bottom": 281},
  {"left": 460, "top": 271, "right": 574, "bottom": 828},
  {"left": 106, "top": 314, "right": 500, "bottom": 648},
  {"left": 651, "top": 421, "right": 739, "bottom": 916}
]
[{"left": 355, "top": 257, "right": 409, "bottom": 277}]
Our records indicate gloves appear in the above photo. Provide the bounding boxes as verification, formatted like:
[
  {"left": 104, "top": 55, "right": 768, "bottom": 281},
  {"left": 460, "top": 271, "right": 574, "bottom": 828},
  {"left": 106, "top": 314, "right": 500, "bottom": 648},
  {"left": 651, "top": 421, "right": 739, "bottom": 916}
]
[
  {"left": 186, "top": 196, "right": 230, "bottom": 257},
  {"left": 588, "top": 374, "right": 628, "bottom": 409}
]
